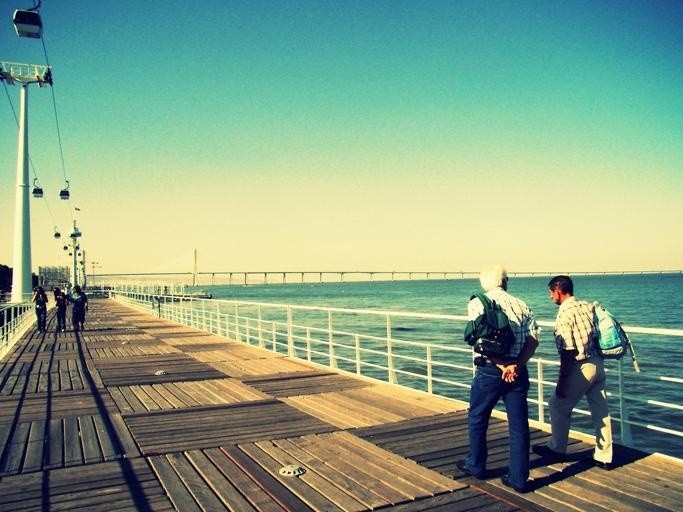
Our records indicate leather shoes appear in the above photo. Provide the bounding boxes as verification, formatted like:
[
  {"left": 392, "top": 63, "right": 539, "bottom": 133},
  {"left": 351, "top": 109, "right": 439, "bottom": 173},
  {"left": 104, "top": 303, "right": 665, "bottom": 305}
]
[
  {"left": 501, "top": 474, "right": 526, "bottom": 493},
  {"left": 576, "top": 452, "right": 612, "bottom": 471},
  {"left": 456, "top": 460, "right": 486, "bottom": 480},
  {"left": 532, "top": 444, "right": 566, "bottom": 462}
]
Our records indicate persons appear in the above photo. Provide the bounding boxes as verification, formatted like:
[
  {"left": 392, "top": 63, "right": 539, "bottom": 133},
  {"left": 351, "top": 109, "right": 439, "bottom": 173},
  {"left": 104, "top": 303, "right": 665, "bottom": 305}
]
[
  {"left": 530, "top": 274, "right": 614, "bottom": 471},
  {"left": 63, "top": 285, "right": 88, "bottom": 331},
  {"left": 455, "top": 261, "right": 539, "bottom": 493},
  {"left": 52, "top": 287, "right": 66, "bottom": 332},
  {"left": 31, "top": 285, "right": 48, "bottom": 333}
]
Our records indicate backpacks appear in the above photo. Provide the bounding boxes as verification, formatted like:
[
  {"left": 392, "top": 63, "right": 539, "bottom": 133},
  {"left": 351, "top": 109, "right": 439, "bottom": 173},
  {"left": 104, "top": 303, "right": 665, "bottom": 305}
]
[
  {"left": 589, "top": 299, "right": 628, "bottom": 361},
  {"left": 464, "top": 292, "right": 515, "bottom": 356}
]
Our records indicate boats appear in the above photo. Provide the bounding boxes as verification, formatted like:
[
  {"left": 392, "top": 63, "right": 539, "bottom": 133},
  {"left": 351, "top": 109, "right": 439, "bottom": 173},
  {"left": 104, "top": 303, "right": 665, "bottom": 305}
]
[{"left": 192, "top": 290, "right": 211, "bottom": 299}]
